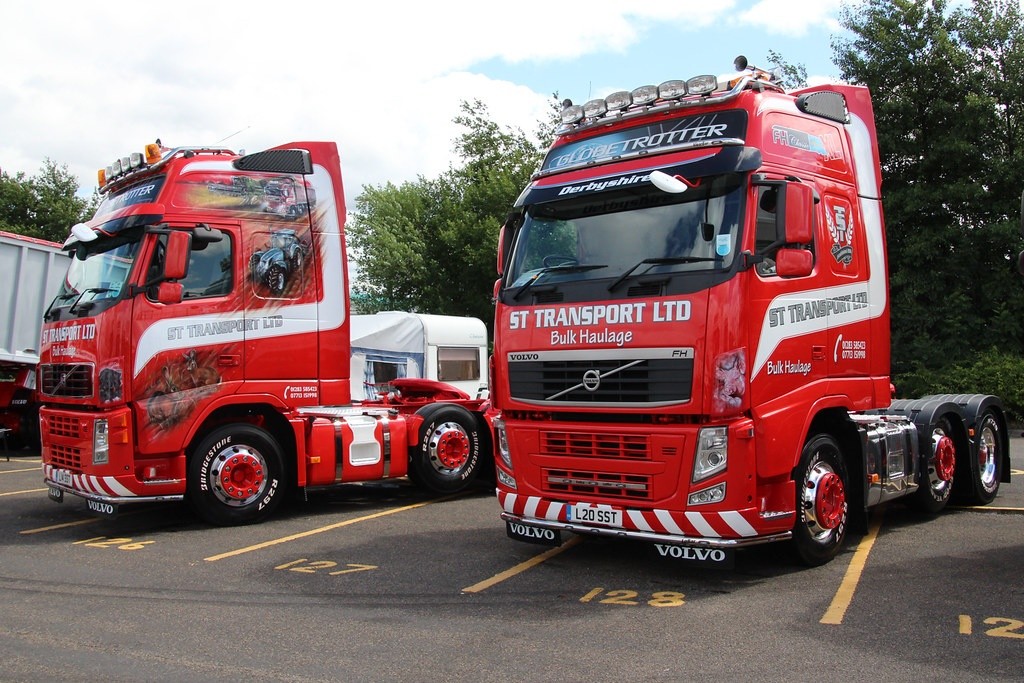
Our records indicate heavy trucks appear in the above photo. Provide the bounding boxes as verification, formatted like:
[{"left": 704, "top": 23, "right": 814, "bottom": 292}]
[
  {"left": 487, "top": 54, "right": 1014, "bottom": 566},
  {"left": 35, "top": 143, "right": 500, "bottom": 526}
]
